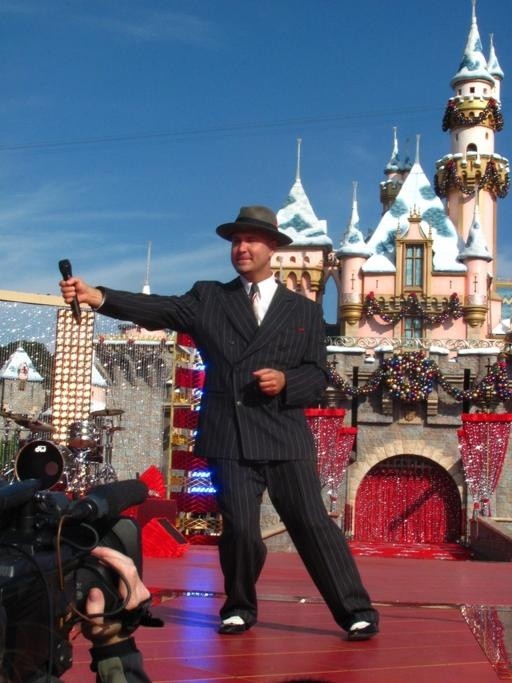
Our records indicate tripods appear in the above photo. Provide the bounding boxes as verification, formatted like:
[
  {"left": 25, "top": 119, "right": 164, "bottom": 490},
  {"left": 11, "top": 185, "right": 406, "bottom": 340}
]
[{"left": 3, "top": 419, "right": 118, "bottom": 499}]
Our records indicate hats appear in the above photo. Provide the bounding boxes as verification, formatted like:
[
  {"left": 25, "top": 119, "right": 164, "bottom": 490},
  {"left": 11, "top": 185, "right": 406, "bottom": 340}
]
[{"left": 217, "top": 206, "right": 291, "bottom": 246}]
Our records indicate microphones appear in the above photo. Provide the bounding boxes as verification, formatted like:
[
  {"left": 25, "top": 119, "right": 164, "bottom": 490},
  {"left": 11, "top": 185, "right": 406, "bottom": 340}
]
[{"left": 58, "top": 259, "right": 83, "bottom": 324}]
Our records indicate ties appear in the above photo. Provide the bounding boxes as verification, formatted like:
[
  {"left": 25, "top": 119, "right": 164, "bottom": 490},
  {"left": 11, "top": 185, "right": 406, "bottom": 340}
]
[{"left": 249, "top": 281, "right": 264, "bottom": 326}]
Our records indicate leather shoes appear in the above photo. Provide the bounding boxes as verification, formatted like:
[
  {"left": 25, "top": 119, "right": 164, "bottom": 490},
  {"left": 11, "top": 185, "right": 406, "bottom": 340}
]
[
  {"left": 347, "top": 620, "right": 378, "bottom": 638},
  {"left": 218, "top": 614, "right": 258, "bottom": 633}
]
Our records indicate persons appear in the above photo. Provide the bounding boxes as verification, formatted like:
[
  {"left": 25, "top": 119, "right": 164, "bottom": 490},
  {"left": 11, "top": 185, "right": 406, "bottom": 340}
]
[
  {"left": 58, "top": 205, "right": 382, "bottom": 642},
  {"left": 1, "top": 543, "right": 156, "bottom": 682}
]
